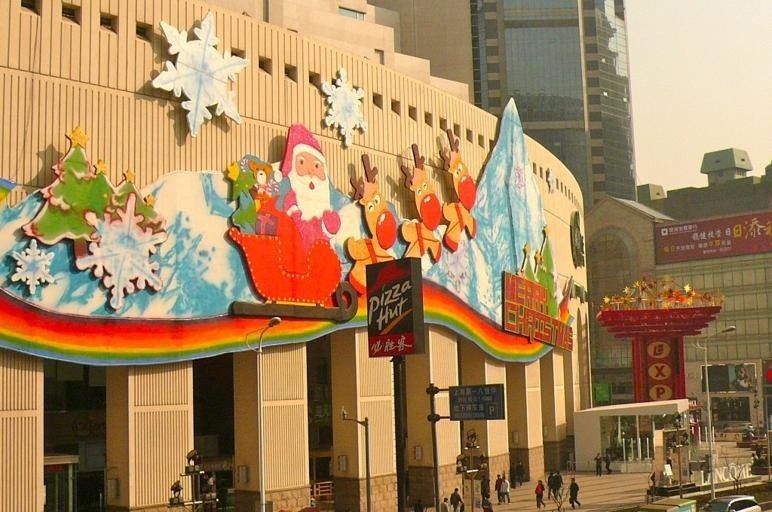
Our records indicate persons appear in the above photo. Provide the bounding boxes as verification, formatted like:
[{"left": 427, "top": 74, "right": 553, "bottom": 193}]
[
  {"left": 594, "top": 453, "right": 603, "bottom": 477},
  {"left": 414, "top": 499, "right": 423, "bottom": 512},
  {"left": 606, "top": 450, "right": 612, "bottom": 474},
  {"left": 450, "top": 488, "right": 463, "bottom": 512},
  {"left": 441, "top": 497, "right": 449, "bottom": 512},
  {"left": 480, "top": 461, "right": 581, "bottom": 512}
]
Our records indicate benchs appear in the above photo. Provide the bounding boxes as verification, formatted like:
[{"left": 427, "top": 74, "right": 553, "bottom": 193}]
[
  {"left": 314, "top": 481, "right": 334, "bottom": 499},
  {"left": 646, "top": 484, "right": 700, "bottom": 503}
]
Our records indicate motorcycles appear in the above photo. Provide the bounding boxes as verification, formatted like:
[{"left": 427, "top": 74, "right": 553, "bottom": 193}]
[{"left": 741, "top": 427, "right": 754, "bottom": 441}]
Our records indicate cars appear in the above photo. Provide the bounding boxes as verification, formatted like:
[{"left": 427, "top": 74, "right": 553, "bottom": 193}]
[
  {"left": 698, "top": 494, "right": 763, "bottom": 512},
  {"left": 725, "top": 422, "right": 752, "bottom": 433}
]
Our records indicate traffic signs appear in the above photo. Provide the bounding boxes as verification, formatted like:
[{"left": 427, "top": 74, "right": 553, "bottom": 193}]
[{"left": 450, "top": 383, "right": 506, "bottom": 421}]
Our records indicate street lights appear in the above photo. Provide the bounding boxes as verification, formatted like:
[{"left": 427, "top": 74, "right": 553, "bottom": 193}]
[
  {"left": 245, "top": 314, "right": 283, "bottom": 512},
  {"left": 740, "top": 361, "right": 760, "bottom": 435},
  {"left": 456, "top": 431, "right": 488, "bottom": 511},
  {"left": 168, "top": 449, "right": 221, "bottom": 512},
  {"left": 688, "top": 324, "right": 736, "bottom": 501},
  {"left": 340, "top": 406, "right": 372, "bottom": 512}
]
